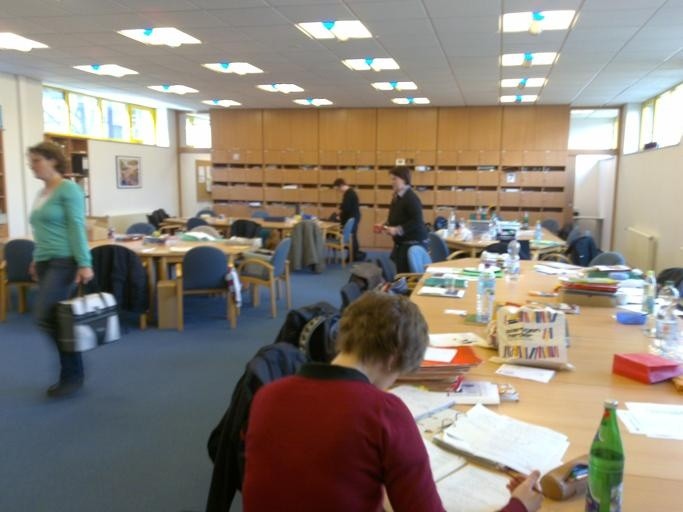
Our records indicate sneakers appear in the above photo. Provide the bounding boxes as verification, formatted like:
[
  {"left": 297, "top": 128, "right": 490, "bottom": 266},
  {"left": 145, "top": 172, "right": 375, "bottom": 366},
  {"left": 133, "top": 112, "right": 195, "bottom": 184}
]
[{"left": 48, "top": 377, "right": 83, "bottom": 397}]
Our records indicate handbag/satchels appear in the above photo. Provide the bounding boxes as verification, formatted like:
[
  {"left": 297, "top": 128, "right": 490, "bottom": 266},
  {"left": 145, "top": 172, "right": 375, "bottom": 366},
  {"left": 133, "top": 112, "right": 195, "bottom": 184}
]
[
  {"left": 489, "top": 305, "right": 576, "bottom": 371},
  {"left": 56, "top": 292, "right": 122, "bottom": 353},
  {"left": 613, "top": 353, "right": 683, "bottom": 385}
]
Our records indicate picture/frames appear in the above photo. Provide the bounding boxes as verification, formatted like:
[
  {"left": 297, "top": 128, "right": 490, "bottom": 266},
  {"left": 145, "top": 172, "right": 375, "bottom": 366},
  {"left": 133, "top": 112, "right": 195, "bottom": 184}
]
[{"left": 115, "top": 155, "right": 143, "bottom": 189}]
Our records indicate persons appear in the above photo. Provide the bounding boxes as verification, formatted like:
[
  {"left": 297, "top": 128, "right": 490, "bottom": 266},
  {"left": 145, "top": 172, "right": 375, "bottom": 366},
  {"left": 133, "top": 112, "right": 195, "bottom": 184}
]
[
  {"left": 27, "top": 141, "right": 95, "bottom": 398},
  {"left": 242, "top": 289, "right": 546, "bottom": 511},
  {"left": 333, "top": 178, "right": 361, "bottom": 261},
  {"left": 374, "top": 166, "right": 430, "bottom": 273}
]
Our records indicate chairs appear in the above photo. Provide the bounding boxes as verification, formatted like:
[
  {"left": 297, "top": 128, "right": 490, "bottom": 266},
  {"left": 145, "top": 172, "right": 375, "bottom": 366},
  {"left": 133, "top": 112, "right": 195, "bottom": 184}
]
[
  {"left": 405, "top": 214, "right": 451, "bottom": 275},
  {"left": 544, "top": 216, "right": 625, "bottom": 266},
  {"left": 175, "top": 243, "right": 238, "bottom": 332},
  {"left": 235, "top": 236, "right": 293, "bottom": 320},
  {"left": 324, "top": 216, "right": 356, "bottom": 272},
  {"left": 0, "top": 237, "right": 39, "bottom": 324},
  {"left": 77, "top": 243, "right": 150, "bottom": 332},
  {"left": 124, "top": 207, "right": 271, "bottom": 239}
]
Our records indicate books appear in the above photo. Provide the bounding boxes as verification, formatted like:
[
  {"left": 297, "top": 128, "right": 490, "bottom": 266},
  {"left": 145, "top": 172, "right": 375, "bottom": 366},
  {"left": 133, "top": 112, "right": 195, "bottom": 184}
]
[
  {"left": 559, "top": 277, "right": 619, "bottom": 308},
  {"left": 418, "top": 279, "right": 467, "bottom": 298},
  {"left": 390, "top": 385, "right": 570, "bottom": 510},
  {"left": 397, "top": 347, "right": 484, "bottom": 382}
]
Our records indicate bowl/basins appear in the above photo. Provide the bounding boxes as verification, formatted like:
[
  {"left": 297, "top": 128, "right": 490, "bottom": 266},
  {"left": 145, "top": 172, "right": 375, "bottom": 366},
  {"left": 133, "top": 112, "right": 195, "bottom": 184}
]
[{"left": 617, "top": 310, "right": 648, "bottom": 323}]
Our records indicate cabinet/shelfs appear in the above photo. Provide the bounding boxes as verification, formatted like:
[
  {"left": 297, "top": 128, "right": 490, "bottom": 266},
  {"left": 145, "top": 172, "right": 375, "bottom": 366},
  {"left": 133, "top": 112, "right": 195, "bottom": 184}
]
[
  {"left": 44, "top": 132, "right": 92, "bottom": 218},
  {"left": 262, "top": 107, "right": 319, "bottom": 225},
  {"left": 432, "top": 105, "right": 501, "bottom": 227},
  {"left": 316, "top": 106, "right": 375, "bottom": 249},
  {"left": 497, "top": 102, "right": 571, "bottom": 233},
  {"left": 208, "top": 107, "right": 263, "bottom": 217},
  {"left": 376, "top": 104, "right": 438, "bottom": 251}
]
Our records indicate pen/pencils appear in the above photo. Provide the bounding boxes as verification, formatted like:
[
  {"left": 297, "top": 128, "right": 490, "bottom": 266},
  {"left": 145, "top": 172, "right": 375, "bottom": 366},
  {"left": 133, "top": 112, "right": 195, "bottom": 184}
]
[{"left": 501, "top": 469, "right": 545, "bottom": 496}]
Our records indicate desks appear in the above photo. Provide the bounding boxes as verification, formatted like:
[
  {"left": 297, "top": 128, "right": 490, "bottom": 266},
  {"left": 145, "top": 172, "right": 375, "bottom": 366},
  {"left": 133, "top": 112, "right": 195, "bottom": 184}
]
[
  {"left": 434, "top": 221, "right": 569, "bottom": 255},
  {"left": 392, "top": 255, "right": 682, "bottom": 511},
  {"left": 163, "top": 214, "right": 343, "bottom": 236},
  {"left": 83, "top": 231, "right": 253, "bottom": 267}
]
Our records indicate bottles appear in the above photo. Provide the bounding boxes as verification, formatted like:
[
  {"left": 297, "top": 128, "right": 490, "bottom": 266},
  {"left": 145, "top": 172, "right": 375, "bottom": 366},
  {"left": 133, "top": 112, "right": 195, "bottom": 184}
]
[
  {"left": 507, "top": 238, "right": 521, "bottom": 283},
  {"left": 655, "top": 281, "right": 680, "bottom": 353},
  {"left": 447, "top": 211, "right": 541, "bottom": 241},
  {"left": 583, "top": 398, "right": 625, "bottom": 512},
  {"left": 642, "top": 270, "right": 656, "bottom": 313},
  {"left": 476, "top": 263, "right": 496, "bottom": 323}
]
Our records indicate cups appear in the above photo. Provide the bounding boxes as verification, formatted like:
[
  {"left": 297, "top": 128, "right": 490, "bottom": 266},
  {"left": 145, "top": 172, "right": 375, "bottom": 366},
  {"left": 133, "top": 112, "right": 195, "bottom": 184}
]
[{"left": 541, "top": 454, "right": 588, "bottom": 502}]
